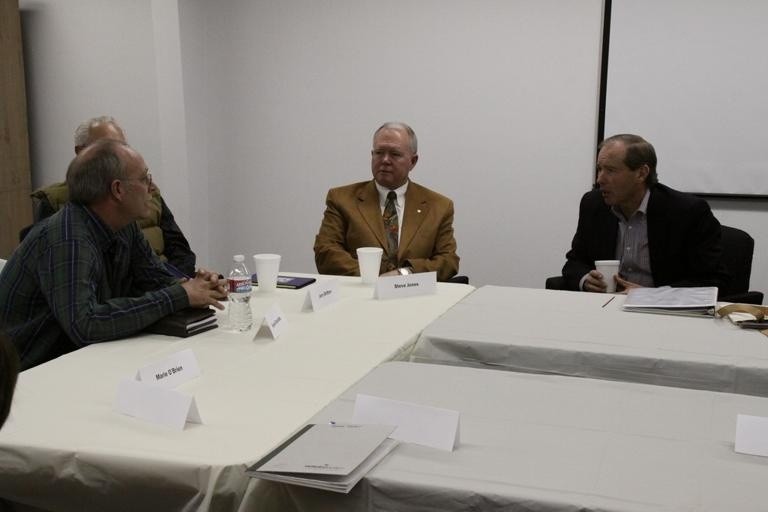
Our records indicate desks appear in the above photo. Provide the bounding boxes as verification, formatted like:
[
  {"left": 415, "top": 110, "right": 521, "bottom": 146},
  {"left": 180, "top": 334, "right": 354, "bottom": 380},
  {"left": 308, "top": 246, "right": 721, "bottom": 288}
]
[
  {"left": 409, "top": 284, "right": 768, "bottom": 397},
  {"left": 281, "top": 361, "right": 768, "bottom": 512},
  {"left": 0, "top": 271, "right": 478, "bottom": 512}
]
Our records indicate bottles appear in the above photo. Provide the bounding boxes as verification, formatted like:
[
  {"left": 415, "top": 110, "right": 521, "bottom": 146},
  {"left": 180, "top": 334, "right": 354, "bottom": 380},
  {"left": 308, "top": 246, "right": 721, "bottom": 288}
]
[{"left": 226, "top": 254, "right": 252, "bottom": 332}]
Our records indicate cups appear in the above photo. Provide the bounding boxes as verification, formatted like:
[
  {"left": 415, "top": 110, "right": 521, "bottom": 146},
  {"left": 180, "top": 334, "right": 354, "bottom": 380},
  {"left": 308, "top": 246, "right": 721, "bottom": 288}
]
[
  {"left": 357, "top": 247, "right": 383, "bottom": 284},
  {"left": 595, "top": 260, "right": 620, "bottom": 293},
  {"left": 253, "top": 254, "right": 281, "bottom": 289}
]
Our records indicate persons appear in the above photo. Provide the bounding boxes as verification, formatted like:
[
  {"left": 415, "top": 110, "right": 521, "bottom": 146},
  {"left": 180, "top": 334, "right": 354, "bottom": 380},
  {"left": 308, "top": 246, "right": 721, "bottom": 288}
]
[
  {"left": 32, "top": 118, "right": 196, "bottom": 278},
  {"left": 562, "top": 134, "right": 723, "bottom": 294},
  {"left": 314, "top": 121, "right": 460, "bottom": 281},
  {"left": 0, "top": 138, "right": 229, "bottom": 372}
]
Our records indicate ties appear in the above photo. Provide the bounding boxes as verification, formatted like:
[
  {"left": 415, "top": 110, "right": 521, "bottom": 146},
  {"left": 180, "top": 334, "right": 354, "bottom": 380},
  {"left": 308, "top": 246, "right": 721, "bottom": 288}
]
[{"left": 383, "top": 191, "right": 398, "bottom": 266}]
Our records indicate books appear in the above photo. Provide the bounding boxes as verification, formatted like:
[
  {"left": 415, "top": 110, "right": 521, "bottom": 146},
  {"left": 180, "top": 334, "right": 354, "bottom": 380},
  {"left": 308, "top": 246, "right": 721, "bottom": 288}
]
[
  {"left": 624, "top": 286, "right": 718, "bottom": 318},
  {"left": 244, "top": 424, "right": 401, "bottom": 495},
  {"left": 153, "top": 309, "right": 218, "bottom": 338},
  {"left": 251, "top": 273, "right": 314, "bottom": 290}
]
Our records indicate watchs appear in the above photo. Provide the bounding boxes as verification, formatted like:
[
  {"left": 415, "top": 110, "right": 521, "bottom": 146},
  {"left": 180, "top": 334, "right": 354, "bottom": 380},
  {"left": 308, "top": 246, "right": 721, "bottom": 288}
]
[{"left": 398, "top": 267, "right": 409, "bottom": 276}]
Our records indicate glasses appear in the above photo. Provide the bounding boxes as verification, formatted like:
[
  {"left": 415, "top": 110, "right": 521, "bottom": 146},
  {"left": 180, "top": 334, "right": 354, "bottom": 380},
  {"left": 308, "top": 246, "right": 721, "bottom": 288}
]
[{"left": 123, "top": 174, "right": 152, "bottom": 185}]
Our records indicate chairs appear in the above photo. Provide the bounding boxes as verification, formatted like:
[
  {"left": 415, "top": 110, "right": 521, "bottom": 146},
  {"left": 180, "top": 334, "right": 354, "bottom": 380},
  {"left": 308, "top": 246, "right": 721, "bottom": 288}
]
[{"left": 545, "top": 225, "right": 765, "bottom": 304}]
